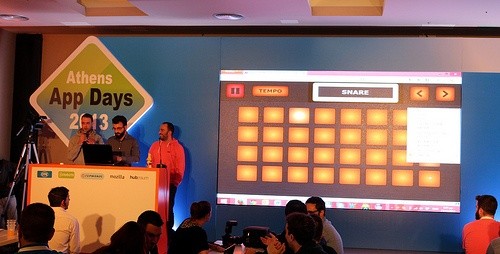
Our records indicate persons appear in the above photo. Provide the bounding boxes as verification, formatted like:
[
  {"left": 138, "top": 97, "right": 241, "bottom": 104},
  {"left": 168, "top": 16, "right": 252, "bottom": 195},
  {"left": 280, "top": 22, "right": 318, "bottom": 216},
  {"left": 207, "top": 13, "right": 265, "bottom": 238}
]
[
  {"left": 0, "top": 166, "right": 500, "bottom": 254},
  {"left": 67, "top": 113, "right": 104, "bottom": 165},
  {"left": 101, "top": 116, "right": 140, "bottom": 167},
  {"left": 147, "top": 122, "right": 186, "bottom": 230}
]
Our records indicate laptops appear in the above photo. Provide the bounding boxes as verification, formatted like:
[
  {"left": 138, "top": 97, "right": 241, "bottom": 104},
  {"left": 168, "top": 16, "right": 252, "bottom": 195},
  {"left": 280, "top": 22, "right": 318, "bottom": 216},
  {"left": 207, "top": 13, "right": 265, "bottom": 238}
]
[{"left": 82, "top": 143, "right": 116, "bottom": 166}]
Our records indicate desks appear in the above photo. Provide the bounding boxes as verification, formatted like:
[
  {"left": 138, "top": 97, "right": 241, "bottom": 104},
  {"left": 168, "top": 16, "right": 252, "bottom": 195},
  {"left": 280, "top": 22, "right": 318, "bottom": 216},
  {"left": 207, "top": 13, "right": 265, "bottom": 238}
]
[
  {"left": 27, "top": 164, "right": 171, "bottom": 254},
  {"left": 206, "top": 243, "right": 264, "bottom": 254},
  {"left": 0, "top": 229, "right": 19, "bottom": 254}
]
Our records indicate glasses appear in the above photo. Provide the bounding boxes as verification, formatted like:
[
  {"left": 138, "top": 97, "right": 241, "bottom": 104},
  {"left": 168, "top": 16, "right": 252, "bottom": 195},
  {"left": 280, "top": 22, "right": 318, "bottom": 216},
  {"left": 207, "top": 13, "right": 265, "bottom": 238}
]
[
  {"left": 112, "top": 126, "right": 125, "bottom": 131},
  {"left": 306, "top": 210, "right": 318, "bottom": 215}
]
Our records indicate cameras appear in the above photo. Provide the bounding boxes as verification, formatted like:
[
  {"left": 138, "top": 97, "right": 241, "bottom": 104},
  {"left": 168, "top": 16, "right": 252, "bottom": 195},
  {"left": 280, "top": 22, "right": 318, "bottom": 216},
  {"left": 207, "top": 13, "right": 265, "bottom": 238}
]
[{"left": 222, "top": 220, "right": 243, "bottom": 249}]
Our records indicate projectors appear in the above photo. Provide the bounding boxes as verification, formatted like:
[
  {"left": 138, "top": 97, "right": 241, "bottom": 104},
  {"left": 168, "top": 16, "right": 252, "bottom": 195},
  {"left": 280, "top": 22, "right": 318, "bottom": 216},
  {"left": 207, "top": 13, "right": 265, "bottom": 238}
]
[{"left": 243, "top": 226, "right": 270, "bottom": 248}]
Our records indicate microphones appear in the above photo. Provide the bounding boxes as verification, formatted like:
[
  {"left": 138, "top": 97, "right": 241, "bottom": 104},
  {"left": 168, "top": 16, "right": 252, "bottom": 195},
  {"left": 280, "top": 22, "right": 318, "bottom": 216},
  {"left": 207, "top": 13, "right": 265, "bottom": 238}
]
[{"left": 83, "top": 130, "right": 87, "bottom": 136}]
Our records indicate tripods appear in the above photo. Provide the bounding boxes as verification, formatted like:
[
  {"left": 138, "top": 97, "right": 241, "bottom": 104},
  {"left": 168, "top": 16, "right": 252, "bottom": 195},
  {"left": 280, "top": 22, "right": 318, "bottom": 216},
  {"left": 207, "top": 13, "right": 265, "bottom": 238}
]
[{"left": 3, "top": 135, "right": 40, "bottom": 224}]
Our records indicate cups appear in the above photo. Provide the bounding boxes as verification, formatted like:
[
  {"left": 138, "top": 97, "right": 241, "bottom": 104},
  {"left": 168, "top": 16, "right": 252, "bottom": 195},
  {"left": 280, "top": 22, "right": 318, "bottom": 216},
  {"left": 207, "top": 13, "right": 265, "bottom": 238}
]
[{"left": 6, "top": 220, "right": 17, "bottom": 236}]
